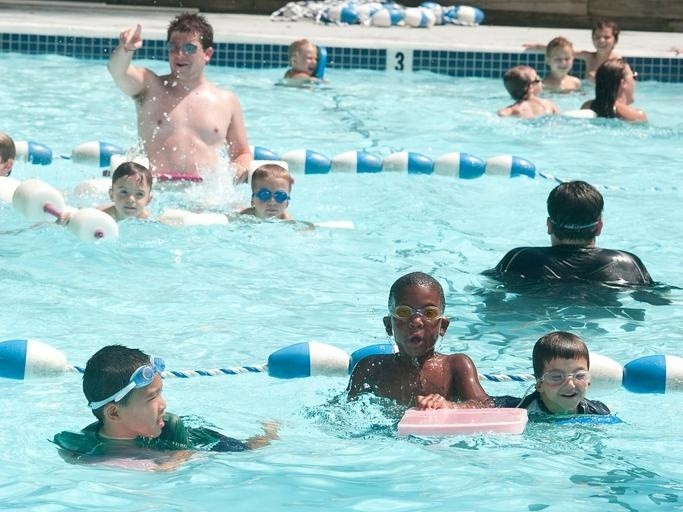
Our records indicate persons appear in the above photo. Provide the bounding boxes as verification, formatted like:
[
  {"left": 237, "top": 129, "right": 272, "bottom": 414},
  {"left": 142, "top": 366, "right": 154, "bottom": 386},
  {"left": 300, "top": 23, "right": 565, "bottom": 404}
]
[
  {"left": 237, "top": 163, "right": 300, "bottom": 224},
  {"left": 106, "top": 10, "right": 252, "bottom": 184},
  {"left": 341, "top": 270, "right": 495, "bottom": 411},
  {"left": 493, "top": 65, "right": 558, "bottom": 118},
  {"left": 49, "top": 342, "right": 280, "bottom": 472},
  {"left": 283, "top": 39, "right": 329, "bottom": 85},
  {"left": 478, "top": 181, "right": 659, "bottom": 286},
  {"left": 0, "top": 131, "right": 18, "bottom": 177},
  {"left": 580, "top": 59, "right": 647, "bottom": 121},
  {"left": 485, "top": 331, "right": 620, "bottom": 425},
  {"left": 669, "top": 46, "right": 682, "bottom": 58},
  {"left": 536, "top": 36, "right": 580, "bottom": 91},
  {"left": 519, "top": 21, "right": 623, "bottom": 85},
  {"left": 98, "top": 162, "right": 154, "bottom": 218}
]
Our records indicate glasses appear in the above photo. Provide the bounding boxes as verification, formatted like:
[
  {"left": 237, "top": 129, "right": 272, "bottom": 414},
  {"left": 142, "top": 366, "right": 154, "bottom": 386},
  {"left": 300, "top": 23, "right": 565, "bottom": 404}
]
[
  {"left": 251, "top": 189, "right": 291, "bottom": 204},
  {"left": 166, "top": 41, "right": 205, "bottom": 57},
  {"left": 529, "top": 75, "right": 541, "bottom": 85},
  {"left": 539, "top": 370, "right": 592, "bottom": 386},
  {"left": 88, "top": 356, "right": 165, "bottom": 410},
  {"left": 388, "top": 303, "right": 443, "bottom": 322}
]
